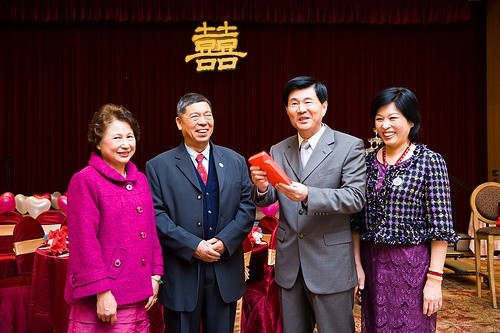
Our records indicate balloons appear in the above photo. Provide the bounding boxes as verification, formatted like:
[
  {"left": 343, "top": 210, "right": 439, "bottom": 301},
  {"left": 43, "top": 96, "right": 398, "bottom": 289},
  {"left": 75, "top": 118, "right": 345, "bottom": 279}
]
[{"left": 0, "top": 192, "right": 67, "bottom": 220}]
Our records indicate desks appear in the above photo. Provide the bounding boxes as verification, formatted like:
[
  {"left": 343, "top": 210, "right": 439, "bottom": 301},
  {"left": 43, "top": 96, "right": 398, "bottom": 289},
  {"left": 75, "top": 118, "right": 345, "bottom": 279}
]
[{"left": 26, "top": 244, "right": 69, "bottom": 333}]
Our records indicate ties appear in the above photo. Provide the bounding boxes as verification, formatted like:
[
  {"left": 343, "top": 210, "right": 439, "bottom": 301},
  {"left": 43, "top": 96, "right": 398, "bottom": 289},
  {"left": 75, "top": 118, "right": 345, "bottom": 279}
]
[
  {"left": 300, "top": 141, "right": 311, "bottom": 172},
  {"left": 195, "top": 154, "right": 207, "bottom": 186}
]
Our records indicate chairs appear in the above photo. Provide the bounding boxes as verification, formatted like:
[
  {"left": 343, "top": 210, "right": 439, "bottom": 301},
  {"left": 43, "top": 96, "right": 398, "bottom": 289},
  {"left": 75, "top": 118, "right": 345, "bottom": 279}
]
[
  {"left": 240, "top": 215, "right": 282, "bottom": 333},
  {"left": 470, "top": 182, "right": 500, "bottom": 308},
  {"left": 0, "top": 208, "right": 65, "bottom": 333}
]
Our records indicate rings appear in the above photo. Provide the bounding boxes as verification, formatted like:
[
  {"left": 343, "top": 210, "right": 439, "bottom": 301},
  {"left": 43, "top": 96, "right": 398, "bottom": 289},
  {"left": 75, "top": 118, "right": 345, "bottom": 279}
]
[{"left": 153, "top": 298, "right": 157, "bottom": 302}]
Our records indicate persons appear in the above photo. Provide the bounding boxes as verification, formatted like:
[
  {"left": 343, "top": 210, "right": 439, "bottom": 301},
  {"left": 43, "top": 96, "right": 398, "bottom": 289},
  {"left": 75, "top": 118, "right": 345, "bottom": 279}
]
[
  {"left": 144, "top": 92, "right": 257, "bottom": 333},
  {"left": 250, "top": 74, "right": 368, "bottom": 332},
  {"left": 64, "top": 105, "right": 165, "bottom": 333},
  {"left": 350, "top": 87, "right": 461, "bottom": 333}
]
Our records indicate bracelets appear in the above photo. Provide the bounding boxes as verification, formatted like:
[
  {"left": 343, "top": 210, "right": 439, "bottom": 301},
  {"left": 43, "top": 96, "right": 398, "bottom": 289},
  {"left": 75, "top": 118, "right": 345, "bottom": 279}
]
[
  {"left": 426, "top": 268, "right": 444, "bottom": 280},
  {"left": 150, "top": 276, "right": 163, "bottom": 284}
]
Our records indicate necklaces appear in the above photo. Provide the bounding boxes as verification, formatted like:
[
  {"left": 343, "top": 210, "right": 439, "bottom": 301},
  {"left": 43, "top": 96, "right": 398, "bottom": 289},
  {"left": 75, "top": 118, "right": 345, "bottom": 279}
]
[{"left": 381, "top": 143, "right": 411, "bottom": 167}]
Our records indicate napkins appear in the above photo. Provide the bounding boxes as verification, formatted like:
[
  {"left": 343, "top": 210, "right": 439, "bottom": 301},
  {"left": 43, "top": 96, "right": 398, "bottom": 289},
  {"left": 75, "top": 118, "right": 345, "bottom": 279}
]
[{"left": 45, "top": 226, "right": 68, "bottom": 256}]
